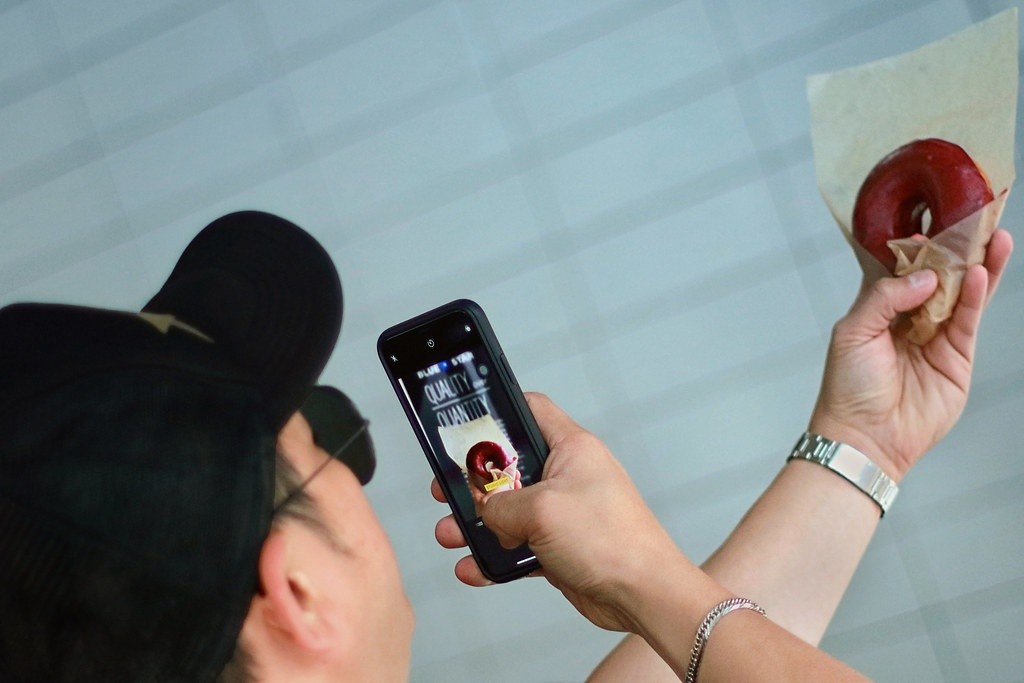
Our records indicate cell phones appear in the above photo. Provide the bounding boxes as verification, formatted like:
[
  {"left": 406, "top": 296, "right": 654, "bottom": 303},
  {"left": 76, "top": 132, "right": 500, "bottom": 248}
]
[{"left": 378, "top": 300, "right": 552, "bottom": 583}]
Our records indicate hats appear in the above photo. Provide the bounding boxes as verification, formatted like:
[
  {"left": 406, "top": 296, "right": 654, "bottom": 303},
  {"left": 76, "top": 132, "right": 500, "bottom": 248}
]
[{"left": 0, "top": 212, "right": 344, "bottom": 683}]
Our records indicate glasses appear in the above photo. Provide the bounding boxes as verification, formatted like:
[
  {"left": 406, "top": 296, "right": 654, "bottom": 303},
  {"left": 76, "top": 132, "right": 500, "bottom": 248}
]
[{"left": 274, "top": 385, "right": 376, "bottom": 517}]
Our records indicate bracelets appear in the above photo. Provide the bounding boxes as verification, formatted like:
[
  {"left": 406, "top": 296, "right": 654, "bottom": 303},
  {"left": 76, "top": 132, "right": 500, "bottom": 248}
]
[
  {"left": 686, "top": 594, "right": 768, "bottom": 683},
  {"left": 784, "top": 434, "right": 899, "bottom": 520}
]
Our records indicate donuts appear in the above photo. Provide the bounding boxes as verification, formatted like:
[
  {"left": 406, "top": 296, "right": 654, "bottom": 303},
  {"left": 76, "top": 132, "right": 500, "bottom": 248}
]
[
  {"left": 853, "top": 138, "right": 996, "bottom": 285},
  {"left": 466, "top": 441, "right": 510, "bottom": 491}
]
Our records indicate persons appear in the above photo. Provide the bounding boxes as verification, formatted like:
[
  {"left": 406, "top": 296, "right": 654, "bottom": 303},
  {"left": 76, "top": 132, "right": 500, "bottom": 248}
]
[{"left": 2, "top": 208, "right": 1014, "bottom": 683}]
